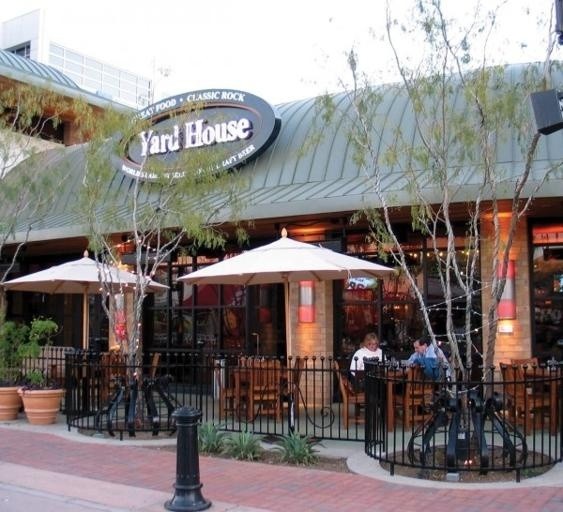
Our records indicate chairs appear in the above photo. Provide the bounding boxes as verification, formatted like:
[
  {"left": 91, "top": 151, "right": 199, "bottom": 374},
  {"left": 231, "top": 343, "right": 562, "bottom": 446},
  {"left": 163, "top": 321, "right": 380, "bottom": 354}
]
[
  {"left": 213, "top": 357, "right": 306, "bottom": 424},
  {"left": 500, "top": 357, "right": 560, "bottom": 439},
  {"left": 392, "top": 363, "right": 436, "bottom": 434},
  {"left": 332, "top": 359, "right": 367, "bottom": 432}
]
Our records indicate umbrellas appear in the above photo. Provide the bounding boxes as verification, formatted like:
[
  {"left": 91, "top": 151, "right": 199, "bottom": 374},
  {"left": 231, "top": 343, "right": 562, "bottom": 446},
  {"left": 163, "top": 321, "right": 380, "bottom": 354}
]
[
  {"left": 174, "top": 228, "right": 393, "bottom": 389},
  {"left": 0, "top": 249, "right": 171, "bottom": 345}
]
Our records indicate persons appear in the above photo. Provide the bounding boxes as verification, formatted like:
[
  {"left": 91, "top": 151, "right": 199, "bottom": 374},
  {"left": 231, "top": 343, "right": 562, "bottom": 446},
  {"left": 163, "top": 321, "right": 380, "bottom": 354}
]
[
  {"left": 404, "top": 337, "right": 452, "bottom": 427},
  {"left": 350, "top": 333, "right": 400, "bottom": 419}
]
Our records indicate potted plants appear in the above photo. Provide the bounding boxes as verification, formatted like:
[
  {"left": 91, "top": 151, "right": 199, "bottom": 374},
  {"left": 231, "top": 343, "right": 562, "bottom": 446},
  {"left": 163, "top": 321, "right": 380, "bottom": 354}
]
[{"left": 0, "top": 319, "right": 65, "bottom": 425}]
